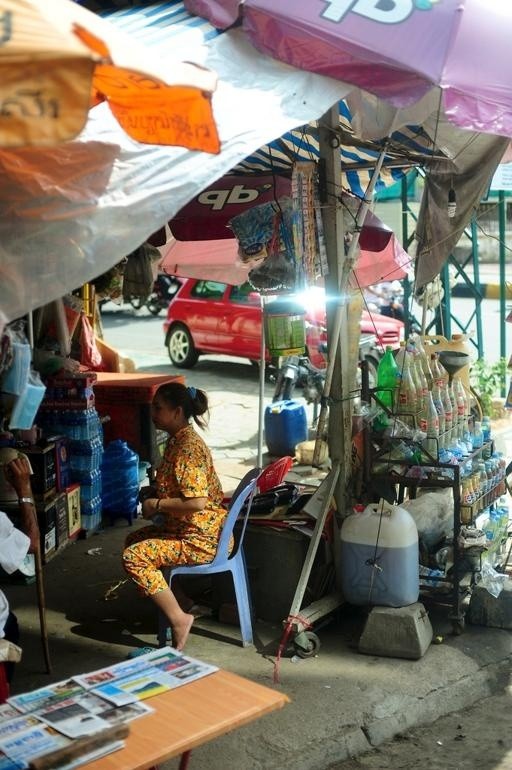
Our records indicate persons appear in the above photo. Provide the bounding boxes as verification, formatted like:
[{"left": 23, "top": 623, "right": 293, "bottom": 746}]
[
  {"left": 0, "top": 457, "right": 41, "bottom": 685},
  {"left": 122, "top": 382, "right": 235, "bottom": 652}
]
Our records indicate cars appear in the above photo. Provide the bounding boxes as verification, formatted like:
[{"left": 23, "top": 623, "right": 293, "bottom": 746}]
[{"left": 158, "top": 275, "right": 406, "bottom": 390}]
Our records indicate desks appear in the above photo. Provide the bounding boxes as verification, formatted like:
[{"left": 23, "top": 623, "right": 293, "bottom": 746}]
[
  {"left": 90, "top": 371, "right": 187, "bottom": 487},
  {"left": 2, "top": 645, "right": 291, "bottom": 769}
]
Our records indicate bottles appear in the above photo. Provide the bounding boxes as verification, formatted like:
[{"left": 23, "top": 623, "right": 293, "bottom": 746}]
[
  {"left": 36, "top": 385, "right": 104, "bottom": 532},
  {"left": 375, "top": 338, "right": 509, "bottom": 594}
]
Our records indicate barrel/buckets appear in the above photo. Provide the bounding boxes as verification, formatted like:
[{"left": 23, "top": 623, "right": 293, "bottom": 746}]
[
  {"left": 102, "top": 440, "right": 139, "bottom": 510},
  {"left": 263, "top": 400, "right": 307, "bottom": 459},
  {"left": 422, "top": 334, "right": 470, "bottom": 391},
  {"left": 263, "top": 313, "right": 306, "bottom": 355},
  {"left": 340, "top": 498, "right": 419, "bottom": 606}
]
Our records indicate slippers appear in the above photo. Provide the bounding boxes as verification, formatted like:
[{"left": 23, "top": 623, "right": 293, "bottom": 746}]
[
  {"left": 157, "top": 628, "right": 172, "bottom": 641},
  {"left": 129, "top": 647, "right": 156, "bottom": 657}
]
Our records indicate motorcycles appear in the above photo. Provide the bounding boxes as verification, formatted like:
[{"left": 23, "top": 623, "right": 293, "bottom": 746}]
[{"left": 374, "top": 290, "right": 425, "bottom": 339}]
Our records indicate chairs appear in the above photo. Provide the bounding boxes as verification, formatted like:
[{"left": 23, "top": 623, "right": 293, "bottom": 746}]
[{"left": 152, "top": 464, "right": 262, "bottom": 653}]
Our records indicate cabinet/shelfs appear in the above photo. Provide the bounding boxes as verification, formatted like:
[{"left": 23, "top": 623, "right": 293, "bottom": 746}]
[{"left": 358, "top": 356, "right": 512, "bottom": 631}]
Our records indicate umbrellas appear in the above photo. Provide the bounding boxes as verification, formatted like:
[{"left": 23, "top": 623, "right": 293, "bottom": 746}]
[
  {"left": 185, "top": 0, "right": 511, "bottom": 467},
  {"left": 145, "top": 172, "right": 413, "bottom": 288}
]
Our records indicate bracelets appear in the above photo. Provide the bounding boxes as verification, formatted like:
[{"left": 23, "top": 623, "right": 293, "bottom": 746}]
[
  {"left": 18, "top": 497, "right": 34, "bottom": 507},
  {"left": 155, "top": 499, "right": 161, "bottom": 514}
]
